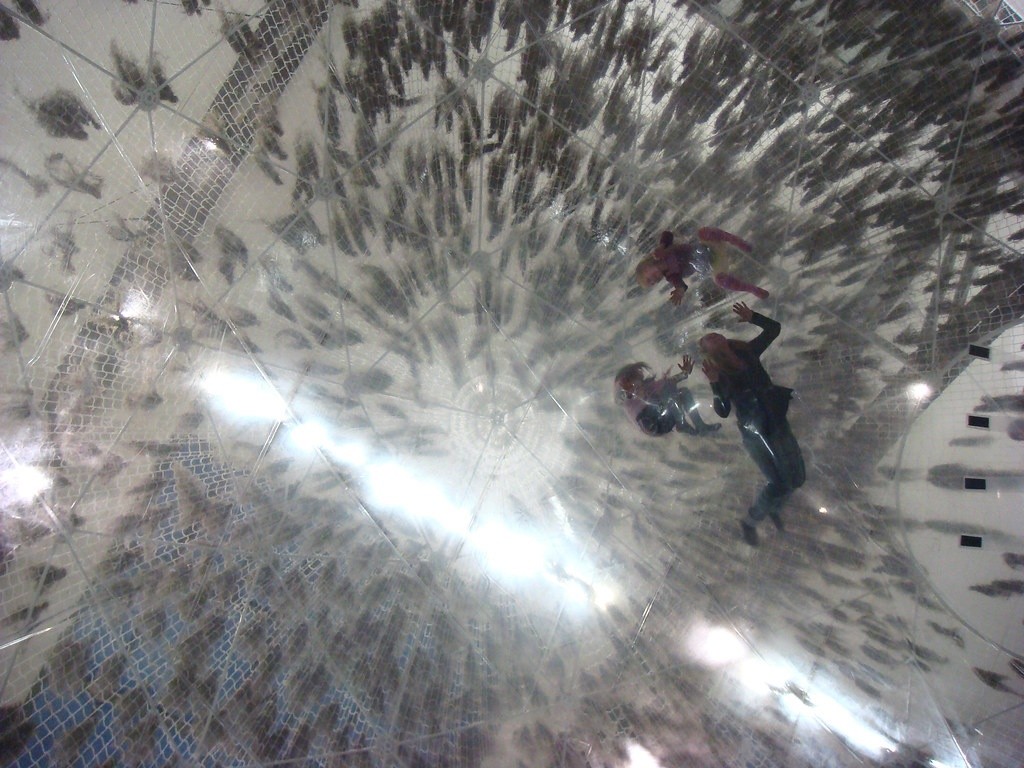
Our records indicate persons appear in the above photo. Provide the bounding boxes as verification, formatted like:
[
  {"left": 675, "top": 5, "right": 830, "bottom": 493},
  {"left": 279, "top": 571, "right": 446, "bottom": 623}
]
[
  {"left": 699, "top": 301, "right": 806, "bottom": 547},
  {"left": 635, "top": 226, "right": 769, "bottom": 306},
  {"left": 614, "top": 355, "right": 721, "bottom": 437}
]
